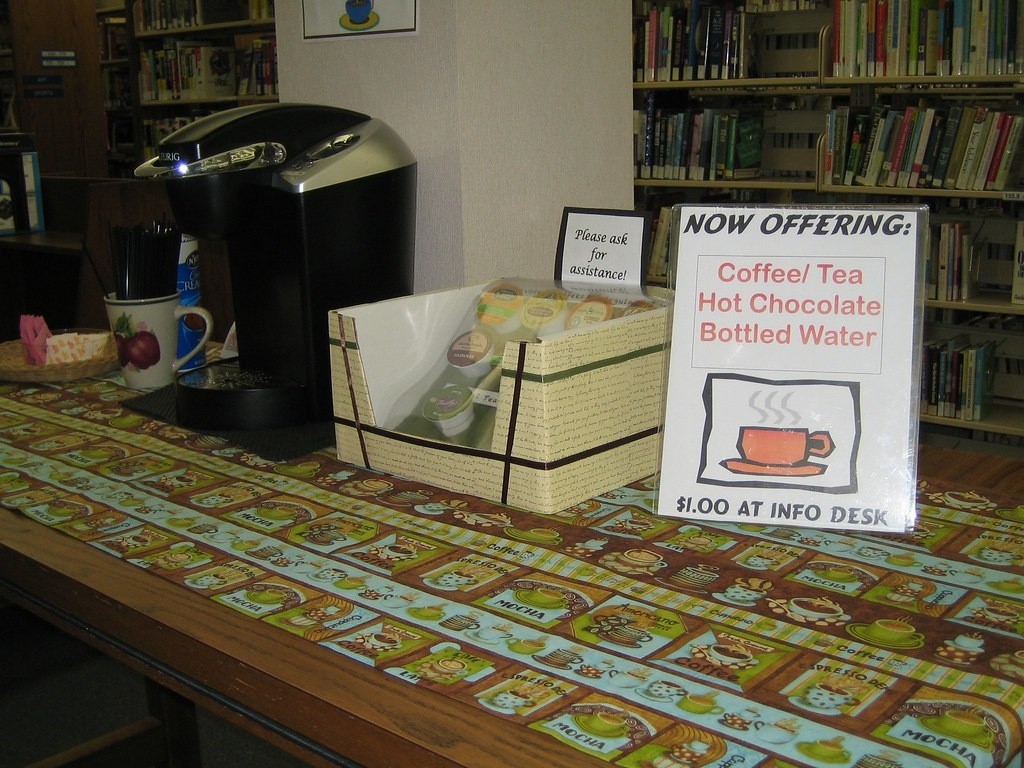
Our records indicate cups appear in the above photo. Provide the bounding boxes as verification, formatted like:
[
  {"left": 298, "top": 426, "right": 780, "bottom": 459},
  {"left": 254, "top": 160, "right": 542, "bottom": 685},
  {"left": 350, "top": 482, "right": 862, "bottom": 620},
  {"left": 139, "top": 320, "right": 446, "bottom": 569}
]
[{"left": 103, "top": 289, "right": 213, "bottom": 391}]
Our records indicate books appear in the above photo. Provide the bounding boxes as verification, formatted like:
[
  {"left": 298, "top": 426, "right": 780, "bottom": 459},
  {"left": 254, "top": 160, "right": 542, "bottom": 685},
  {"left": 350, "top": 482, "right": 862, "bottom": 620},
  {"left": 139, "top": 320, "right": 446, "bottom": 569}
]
[
  {"left": 97, "top": 0, "right": 279, "bottom": 161},
  {"left": 633, "top": 0, "right": 1024, "bottom": 421}
]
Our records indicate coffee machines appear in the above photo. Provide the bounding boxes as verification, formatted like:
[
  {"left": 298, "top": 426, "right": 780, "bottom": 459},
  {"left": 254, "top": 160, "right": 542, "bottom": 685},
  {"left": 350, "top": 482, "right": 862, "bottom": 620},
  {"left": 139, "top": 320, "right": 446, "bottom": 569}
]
[{"left": 133, "top": 101, "right": 417, "bottom": 430}]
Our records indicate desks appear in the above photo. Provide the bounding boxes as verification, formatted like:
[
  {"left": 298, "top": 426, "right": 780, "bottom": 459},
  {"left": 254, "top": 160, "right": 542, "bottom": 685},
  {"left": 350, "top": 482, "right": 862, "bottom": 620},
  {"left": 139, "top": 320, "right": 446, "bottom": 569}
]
[{"left": 0, "top": 323, "right": 1022, "bottom": 765}]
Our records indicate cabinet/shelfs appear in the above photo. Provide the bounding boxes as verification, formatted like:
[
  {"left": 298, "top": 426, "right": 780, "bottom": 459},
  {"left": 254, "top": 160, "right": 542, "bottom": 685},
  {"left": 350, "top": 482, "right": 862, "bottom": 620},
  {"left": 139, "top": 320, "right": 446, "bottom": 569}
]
[
  {"left": 0, "top": 1, "right": 281, "bottom": 179},
  {"left": 632, "top": 0, "right": 1024, "bottom": 432}
]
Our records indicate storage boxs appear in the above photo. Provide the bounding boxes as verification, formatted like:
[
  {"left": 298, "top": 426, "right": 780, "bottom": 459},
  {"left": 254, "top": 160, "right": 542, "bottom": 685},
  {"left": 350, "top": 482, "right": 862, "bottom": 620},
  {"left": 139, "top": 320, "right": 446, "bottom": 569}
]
[
  {"left": 326, "top": 277, "right": 680, "bottom": 516},
  {"left": 0, "top": 134, "right": 46, "bottom": 238}
]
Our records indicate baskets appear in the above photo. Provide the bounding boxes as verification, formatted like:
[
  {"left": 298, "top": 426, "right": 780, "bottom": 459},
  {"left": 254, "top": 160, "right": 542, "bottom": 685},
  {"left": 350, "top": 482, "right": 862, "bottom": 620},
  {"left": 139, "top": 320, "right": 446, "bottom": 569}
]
[{"left": 0, "top": 327, "right": 119, "bottom": 382}]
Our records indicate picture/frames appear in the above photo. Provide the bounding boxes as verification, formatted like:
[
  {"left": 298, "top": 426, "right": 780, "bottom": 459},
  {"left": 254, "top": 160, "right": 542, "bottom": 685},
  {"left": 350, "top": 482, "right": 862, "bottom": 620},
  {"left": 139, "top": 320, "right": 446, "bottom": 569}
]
[{"left": 302, "top": 0, "right": 417, "bottom": 40}]
